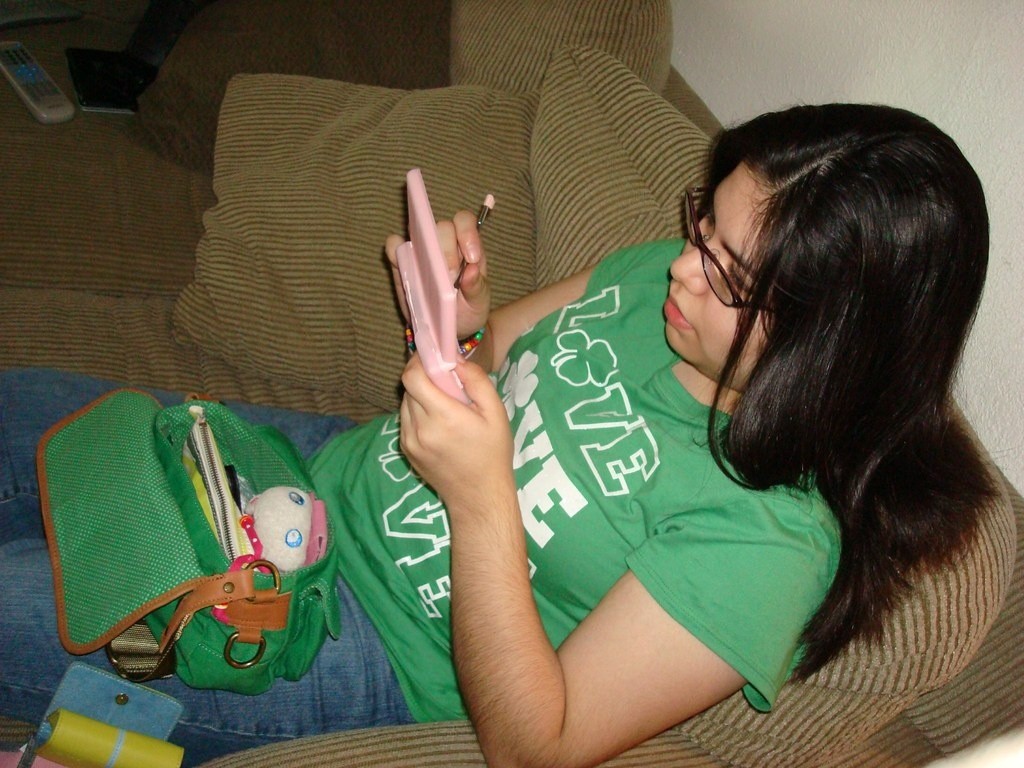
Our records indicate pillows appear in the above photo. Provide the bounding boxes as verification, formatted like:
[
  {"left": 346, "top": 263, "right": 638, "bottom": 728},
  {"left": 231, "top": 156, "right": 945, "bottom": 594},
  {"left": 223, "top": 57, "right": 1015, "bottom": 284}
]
[{"left": 169, "top": 75, "right": 538, "bottom": 413}]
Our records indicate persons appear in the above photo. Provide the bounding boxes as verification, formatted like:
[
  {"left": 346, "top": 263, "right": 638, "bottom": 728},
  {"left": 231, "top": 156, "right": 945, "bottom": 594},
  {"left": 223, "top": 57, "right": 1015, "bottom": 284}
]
[{"left": 0, "top": 104, "right": 997, "bottom": 768}]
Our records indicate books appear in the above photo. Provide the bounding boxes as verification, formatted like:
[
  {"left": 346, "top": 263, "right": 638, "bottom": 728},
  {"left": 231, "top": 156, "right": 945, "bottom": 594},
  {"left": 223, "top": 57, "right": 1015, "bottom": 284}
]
[{"left": 64, "top": 48, "right": 136, "bottom": 115}]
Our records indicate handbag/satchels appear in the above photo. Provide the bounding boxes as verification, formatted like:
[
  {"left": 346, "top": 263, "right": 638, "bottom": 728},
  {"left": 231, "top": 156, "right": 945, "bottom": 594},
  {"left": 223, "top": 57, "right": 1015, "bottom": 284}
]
[{"left": 38, "top": 388, "right": 342, "bottom": 696}]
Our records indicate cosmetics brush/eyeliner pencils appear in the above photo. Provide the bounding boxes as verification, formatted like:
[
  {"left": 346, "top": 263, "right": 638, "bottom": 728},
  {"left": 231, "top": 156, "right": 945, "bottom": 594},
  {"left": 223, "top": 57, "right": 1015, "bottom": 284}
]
[{"left": 453, "top": 194, "right": 496, "bottom": 300}]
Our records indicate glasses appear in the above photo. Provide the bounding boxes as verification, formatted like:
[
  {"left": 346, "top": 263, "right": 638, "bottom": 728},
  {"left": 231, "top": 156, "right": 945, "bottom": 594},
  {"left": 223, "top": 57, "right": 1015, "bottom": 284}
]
[{"left": 685, "top": 187, "right": 775, "bottom": 315}]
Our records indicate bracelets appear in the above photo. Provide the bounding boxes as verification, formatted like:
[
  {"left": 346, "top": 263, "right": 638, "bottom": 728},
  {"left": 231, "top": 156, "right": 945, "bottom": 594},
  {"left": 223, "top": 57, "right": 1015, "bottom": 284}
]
[{"left": 405, "top": 324, "right": 487, "bottom": 360}]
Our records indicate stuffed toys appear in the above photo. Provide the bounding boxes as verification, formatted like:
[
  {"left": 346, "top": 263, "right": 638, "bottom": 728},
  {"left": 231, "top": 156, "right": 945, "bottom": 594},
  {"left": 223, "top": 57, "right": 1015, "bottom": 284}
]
[{"left": 246, "top": 485, "right": 328, "bottom": 574}]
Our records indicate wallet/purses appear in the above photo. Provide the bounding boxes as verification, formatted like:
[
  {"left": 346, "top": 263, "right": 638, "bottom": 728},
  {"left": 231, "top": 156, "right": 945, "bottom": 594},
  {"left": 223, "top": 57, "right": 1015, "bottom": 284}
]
[{"left": 32, "top": 660, "right": 185, "bottom": 768}]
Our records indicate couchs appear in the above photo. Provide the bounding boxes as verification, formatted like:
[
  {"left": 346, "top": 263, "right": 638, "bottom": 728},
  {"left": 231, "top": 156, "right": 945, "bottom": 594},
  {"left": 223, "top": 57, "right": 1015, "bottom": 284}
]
[{"left": 0, "top": 0, "right": 1024, "bottom": 768}]
